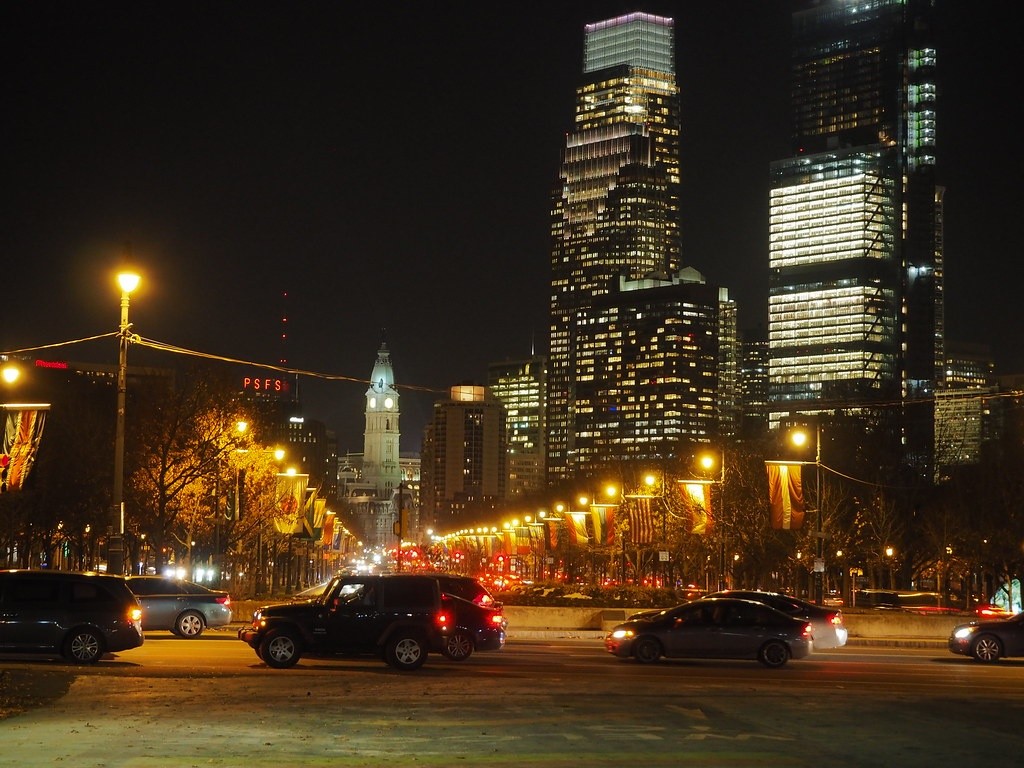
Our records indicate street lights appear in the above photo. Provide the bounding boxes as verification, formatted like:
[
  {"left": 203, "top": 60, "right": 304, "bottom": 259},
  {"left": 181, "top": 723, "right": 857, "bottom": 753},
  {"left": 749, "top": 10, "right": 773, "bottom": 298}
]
[
  {"left": 107, "top": 268, "right": 144, "bottom": 574},
  {"left": 214, "top": 419, "right": 249, "bottom": 592},
  {"left": 579, "top": 495, "right": 595, "bottom": 584},
  {"left": 607, "top": 481, "right": 624, "bottom": 586},
  {"left": 645, "top": 466, "right": 667, "bottom": 588},
  {"left": 430, "top": 501, "right": 571, "bottom": 585},
  {"left": 272, "top": 466, "right": 332, "bottom": 595},
  {"left": 791, "top": 422, "right": 822, "bottom": 606},
  {"left": 700, "top": 448, "right": 726, "bottom": 592},
  {"left": 397, "top": 482, "right": 410, "bottom": 573},
  {"left": 256, "top": 450, "right": 286, "bottom": 595}
]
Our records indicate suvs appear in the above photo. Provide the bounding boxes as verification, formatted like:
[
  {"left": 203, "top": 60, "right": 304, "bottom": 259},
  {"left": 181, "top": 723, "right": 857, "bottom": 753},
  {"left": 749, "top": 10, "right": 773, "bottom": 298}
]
[{"left": 237, "top": 576, "right": 450, "bottom": 671}]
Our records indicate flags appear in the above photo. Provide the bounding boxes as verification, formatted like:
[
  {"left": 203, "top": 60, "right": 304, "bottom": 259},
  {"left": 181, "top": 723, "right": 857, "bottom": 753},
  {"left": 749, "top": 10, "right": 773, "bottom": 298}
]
[
  {"left": 0, "top": 410, "right": 46, "bottom": 493},
  {"left": 679, "top": 484, "right": 713, "bottom": 535},
  {"left": 591, "top": 507, "right": 615, "bottom": 545},
  {"left": 504, "top": 533, "right": 517, "bottom": 554},
  {"left": 566, "top": 515, "right": 589, "bottom": 545},
  {"left": 292, "top": 492, "right": 315, "bottom": 537},
  {"left": 274, "top": 476, "right": 307, "bottom": 533},
  {"left": 226, "top": 468, "right": 246, "bottom": 520},
  {"left": 299, "top": 501, "right": 325, "bottom": 540},
  {"left": 544, "top": 521, "right": 561, "bottom": 550},
  {"left": 767, "top": 465, "right": 805, "bottom": 529},
  {"left": 431, "top": 534, "right": 503, "bottom": 557},
  {"left": 315, "top": 514, "right": 335, "bottom": 545},
  {"left": 515, "top": 529, "right": 531, "bottom": 554},
  {"left": 627, "top": 498, "right": 654, "bottom": 543},
  {"left": 318, "top": 520, "right": 356, "bottom": 553}
]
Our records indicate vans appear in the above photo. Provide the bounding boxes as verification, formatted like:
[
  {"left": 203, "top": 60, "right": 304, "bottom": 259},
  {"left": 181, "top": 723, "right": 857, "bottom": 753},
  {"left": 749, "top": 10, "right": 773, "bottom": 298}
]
[{"left": 0, "top": 567, "right": 144, "bottom": 665}]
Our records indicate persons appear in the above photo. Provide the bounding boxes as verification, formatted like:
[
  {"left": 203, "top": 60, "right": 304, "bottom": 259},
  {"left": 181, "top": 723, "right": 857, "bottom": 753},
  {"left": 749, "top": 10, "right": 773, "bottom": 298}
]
[
  {"left": 686, "top": 608, "right": 713, "bottom": 624},
  {"left": 345, "top": 585, "right": 374, "bottom": 605}
]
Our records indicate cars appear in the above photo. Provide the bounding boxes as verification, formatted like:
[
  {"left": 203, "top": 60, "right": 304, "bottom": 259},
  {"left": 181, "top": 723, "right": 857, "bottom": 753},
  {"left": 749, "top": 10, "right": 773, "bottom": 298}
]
[
  {"left": 809, "top": 592, "right": 843, "bottom": 607},
  {"left": 125, "top": 576, "right": 232, "bottom": 639},
  {"left": 947, "top": 604, "right": 1024, "bottom": 662},
  {"left": 344, "top": 574, "right": 507, "bottom": 661},
  {"left": 602, "top": 597, "right": 814, "bottom": 666},
  {"left": 478, "top": 574, "right": 533, "bottom": 588},
  {"left": 626, "top": 591, "right": 849, "bottom": 652}
]
